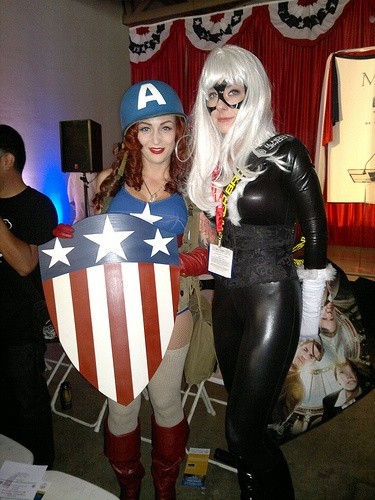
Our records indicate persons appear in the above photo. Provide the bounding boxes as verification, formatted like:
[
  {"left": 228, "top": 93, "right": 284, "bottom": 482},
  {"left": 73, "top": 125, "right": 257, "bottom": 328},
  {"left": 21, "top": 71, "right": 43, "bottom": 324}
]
[
  {"left": 50, "top": 79, "right": 218, "bottom": 500},
  {"left": 319, "top": 270, "right": 342, "bottom": 337},
  {"left": 322, "top": 357, "right": 367, "bottom": 422},
  {"left": 272, "top": 339, "right": 324, "bottom": 436},
  {"left": 0, "top": 125, "right": 57, "bottom": 470},
  {"left": 67, "top": 171, "right": 98, "bottom": 223}
]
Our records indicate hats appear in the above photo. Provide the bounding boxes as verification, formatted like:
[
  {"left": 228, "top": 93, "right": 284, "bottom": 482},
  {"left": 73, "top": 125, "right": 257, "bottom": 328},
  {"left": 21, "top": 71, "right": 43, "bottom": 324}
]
[{"left": 119, "top": 81, "right": 187, "bottom": 132}]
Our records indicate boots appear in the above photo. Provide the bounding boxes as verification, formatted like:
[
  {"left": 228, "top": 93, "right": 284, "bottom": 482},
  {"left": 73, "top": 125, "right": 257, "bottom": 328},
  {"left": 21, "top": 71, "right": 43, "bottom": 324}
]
[
  {"left": 150, "top": 418, "right": 190, "bottom": 500},
  {"left": 102, "top": 417, "right": 145, "bottom": 500}
]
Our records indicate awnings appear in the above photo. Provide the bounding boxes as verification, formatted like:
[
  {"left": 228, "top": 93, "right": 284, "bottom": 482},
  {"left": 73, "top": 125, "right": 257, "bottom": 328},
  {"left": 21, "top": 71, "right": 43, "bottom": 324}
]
[{"left": 189, "top": 43, "right": 337, "bottom": 500}]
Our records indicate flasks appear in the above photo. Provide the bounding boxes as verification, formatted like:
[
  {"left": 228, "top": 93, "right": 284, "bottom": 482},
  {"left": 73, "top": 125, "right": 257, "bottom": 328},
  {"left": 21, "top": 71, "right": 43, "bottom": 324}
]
[{"left": 60, "top": 381, "right": 72, "bottom": 410}]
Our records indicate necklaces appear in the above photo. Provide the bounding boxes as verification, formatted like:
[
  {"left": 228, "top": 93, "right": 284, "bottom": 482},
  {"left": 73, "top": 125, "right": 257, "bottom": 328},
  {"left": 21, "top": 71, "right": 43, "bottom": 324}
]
[{"left": 139, "top": 177, "right": 170, "bottom": 203}]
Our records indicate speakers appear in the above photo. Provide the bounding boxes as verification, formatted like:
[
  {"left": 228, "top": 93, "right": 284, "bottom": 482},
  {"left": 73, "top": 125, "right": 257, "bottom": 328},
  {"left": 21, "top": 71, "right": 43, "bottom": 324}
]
[{"left": 59, "top": 119, "right": 103, "bottom": 173}]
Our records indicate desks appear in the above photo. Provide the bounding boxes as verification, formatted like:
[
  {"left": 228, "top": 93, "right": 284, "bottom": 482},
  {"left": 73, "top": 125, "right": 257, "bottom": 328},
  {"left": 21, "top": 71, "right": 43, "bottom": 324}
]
[{"left": 0, "top": 433, "right": 119, "bottom": 500}]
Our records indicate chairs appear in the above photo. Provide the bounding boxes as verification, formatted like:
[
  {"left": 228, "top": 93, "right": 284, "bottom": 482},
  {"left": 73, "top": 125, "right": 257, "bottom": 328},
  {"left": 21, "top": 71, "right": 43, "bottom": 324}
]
[{"left": 46, "top": 274, "right": 251, "bottom": 480}]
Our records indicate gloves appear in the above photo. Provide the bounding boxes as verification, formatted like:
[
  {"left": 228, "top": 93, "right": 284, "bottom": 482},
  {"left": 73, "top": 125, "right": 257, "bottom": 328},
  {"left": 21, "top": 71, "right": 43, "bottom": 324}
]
[
  {"left": 297, "top": 264, "right": 337, "bottom": 343},
  {"left": 53, "top": 224, "right": 75, "bottom": 239},
  {"left": 179, "top": 247, "right": 211, "bottom": 277}
]
